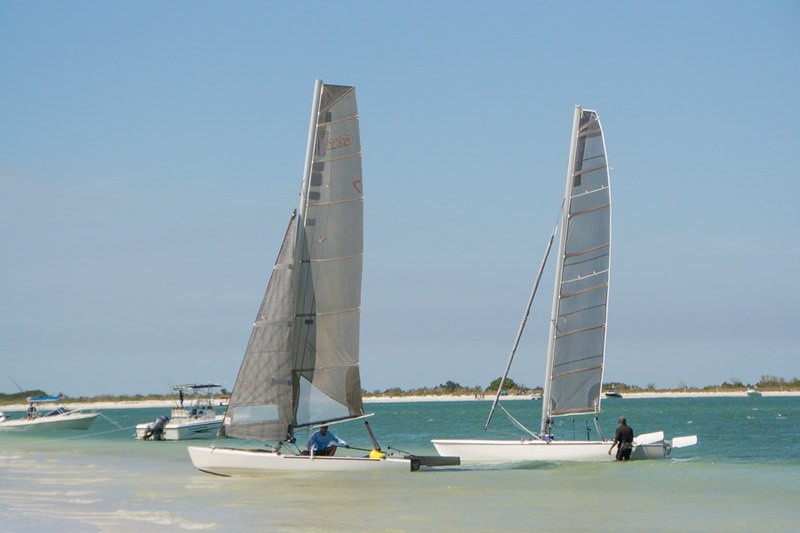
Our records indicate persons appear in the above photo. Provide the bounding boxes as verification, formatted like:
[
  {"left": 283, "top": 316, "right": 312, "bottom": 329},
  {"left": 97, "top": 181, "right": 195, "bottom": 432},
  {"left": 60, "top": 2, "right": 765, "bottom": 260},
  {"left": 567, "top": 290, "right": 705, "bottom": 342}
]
[
  {"left": 26, "top": 402, "right": 36, "bottom": 420},
  {"left": 608, "top": 417, "right": 635, "bottom": 461},
  {"left": 303, "top": 423, "right": 349, "bottom": 457}
]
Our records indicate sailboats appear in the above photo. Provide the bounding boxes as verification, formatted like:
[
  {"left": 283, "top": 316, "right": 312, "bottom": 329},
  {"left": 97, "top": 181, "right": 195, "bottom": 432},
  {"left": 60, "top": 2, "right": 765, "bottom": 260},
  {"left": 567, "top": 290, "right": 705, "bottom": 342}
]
[
  {"left": 181, "top": 79, "right": 459, "bottom": 472},
  {"left": 421, "top": 105, "right": 699, "bottom": 461}
]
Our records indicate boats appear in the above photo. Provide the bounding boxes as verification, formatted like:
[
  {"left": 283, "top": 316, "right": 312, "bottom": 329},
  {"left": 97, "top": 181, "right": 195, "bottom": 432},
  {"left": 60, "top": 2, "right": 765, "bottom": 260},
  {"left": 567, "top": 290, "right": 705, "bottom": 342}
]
[
  {"left": 602, "top": 387, "right": 624, "bottom": 398},
  {"left": 131, "top": 382, "right": 235, "bottom": 443},
  {"left": 746, "top": 390, "right": 762, "bottom": 397},
  {"left": 0, "top": 394, "right": 102, "bottom": 431}
]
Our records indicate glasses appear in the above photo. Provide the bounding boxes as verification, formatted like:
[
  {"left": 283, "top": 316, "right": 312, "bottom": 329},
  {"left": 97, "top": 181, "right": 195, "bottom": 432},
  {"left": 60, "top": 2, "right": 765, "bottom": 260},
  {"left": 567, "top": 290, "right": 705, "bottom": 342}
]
[{"left": 322, "top": 427, "right": 328, "bottom": 429}]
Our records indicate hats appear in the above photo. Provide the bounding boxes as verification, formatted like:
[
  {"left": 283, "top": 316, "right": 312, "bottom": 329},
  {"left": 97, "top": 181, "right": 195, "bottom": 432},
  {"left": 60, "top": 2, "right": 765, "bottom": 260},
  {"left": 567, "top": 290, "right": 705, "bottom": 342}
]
[{"left": 618, "top": 417, "right": 626, "bottom": 424}]
